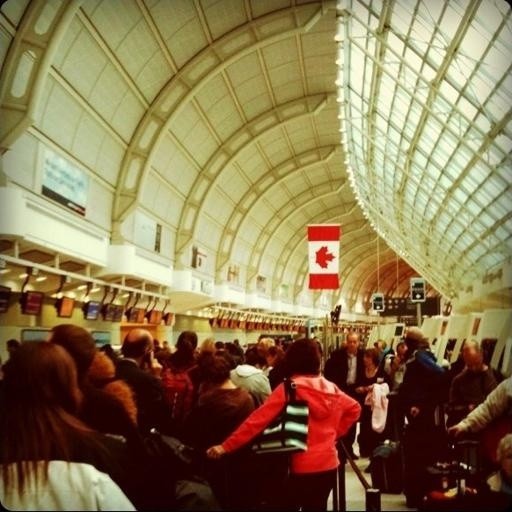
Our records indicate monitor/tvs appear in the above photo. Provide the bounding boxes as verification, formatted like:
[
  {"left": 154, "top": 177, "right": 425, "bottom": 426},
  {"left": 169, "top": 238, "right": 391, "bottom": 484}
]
[
  {"left": 210, "top": 318, "right": 369, "bottom": 334},
  {"left": 393, "top": 324, "right": 404, "bottom": 338},
  {"left": 0, "top": 286, "right": 174, "bottom": 325}
]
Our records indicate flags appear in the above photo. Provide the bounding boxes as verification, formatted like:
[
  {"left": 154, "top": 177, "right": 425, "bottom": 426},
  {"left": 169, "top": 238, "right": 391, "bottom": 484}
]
[{"left": 306, "top": 223, "right": 341, "bottom": 291}]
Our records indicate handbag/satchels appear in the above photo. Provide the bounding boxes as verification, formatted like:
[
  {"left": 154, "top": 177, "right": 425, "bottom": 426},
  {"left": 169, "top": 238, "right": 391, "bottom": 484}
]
[{"left": 248, "top": 376, "right": 310, "bottom": 455}]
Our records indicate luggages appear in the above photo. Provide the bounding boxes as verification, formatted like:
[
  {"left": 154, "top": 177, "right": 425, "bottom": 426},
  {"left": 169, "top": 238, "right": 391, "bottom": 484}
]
[
  {"left": 417, "top": 438, "right": 495, "bottom": 512},
  {"left": 169, "top": 454, "right": 224, "bottom": 512},
  {"left": 368, "top": 390, "right": 404, "bottom": 495}
]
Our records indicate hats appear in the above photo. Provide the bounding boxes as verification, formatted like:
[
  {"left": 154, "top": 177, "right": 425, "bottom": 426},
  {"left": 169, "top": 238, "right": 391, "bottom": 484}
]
[{"left": 401, "top": 326, "right": 430, "bottom": 345}]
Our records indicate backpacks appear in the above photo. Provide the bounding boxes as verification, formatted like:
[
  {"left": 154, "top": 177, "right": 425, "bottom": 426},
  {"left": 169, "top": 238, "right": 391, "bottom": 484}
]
[{"left": 159, "top": 360, "right": 200, "bottom": 424}]
